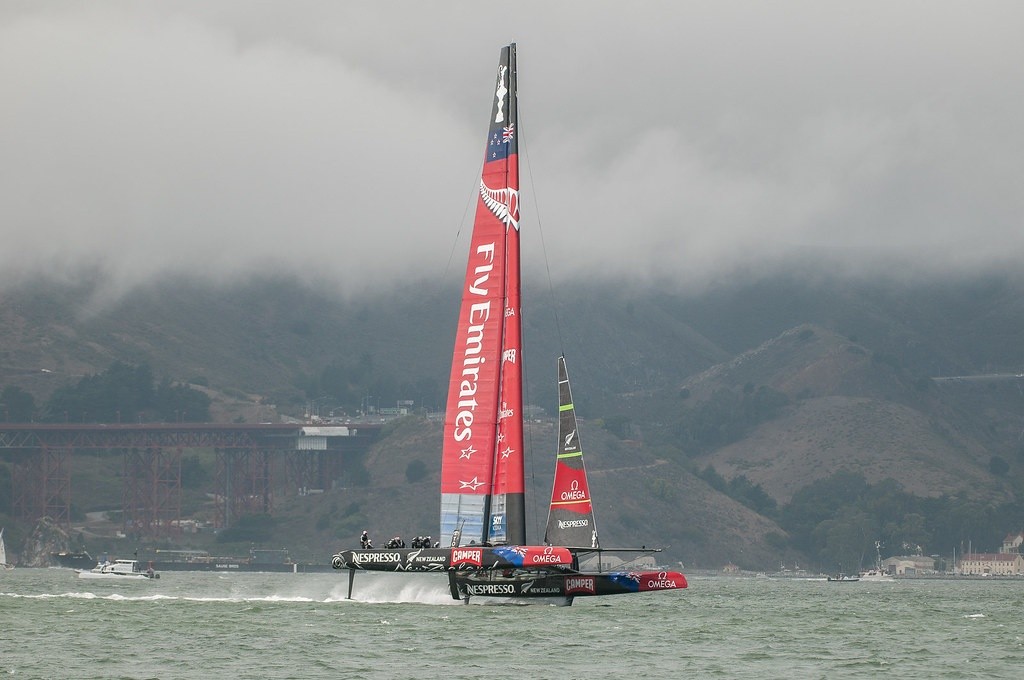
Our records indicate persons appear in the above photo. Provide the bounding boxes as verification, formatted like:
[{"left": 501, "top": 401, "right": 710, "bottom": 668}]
[
  {"left": 360, "top": 531, "right": 374, "bottom": 549},
  {"left": 384, "top": 536, "right": 439, "bottom": 549}
]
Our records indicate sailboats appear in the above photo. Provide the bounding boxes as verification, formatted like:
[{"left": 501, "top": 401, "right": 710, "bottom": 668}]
[{"left": 331, "top": 39, "right": 689, "bottom": 603}]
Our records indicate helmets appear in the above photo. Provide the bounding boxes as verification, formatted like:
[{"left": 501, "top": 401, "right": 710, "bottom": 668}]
[
  {"left": 418, "top": 536, "right": 422, "bottom": 541},
  {"left": 363, "top": 531, "right": 367, "bottom": 535},
  {"left": 427, "top": 536, "right": 431, "bottom": 540}
]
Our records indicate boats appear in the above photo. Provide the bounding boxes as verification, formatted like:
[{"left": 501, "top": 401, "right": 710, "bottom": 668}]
[{"left": 90, "top": 558, "right": 162, "bottom": 580}]
